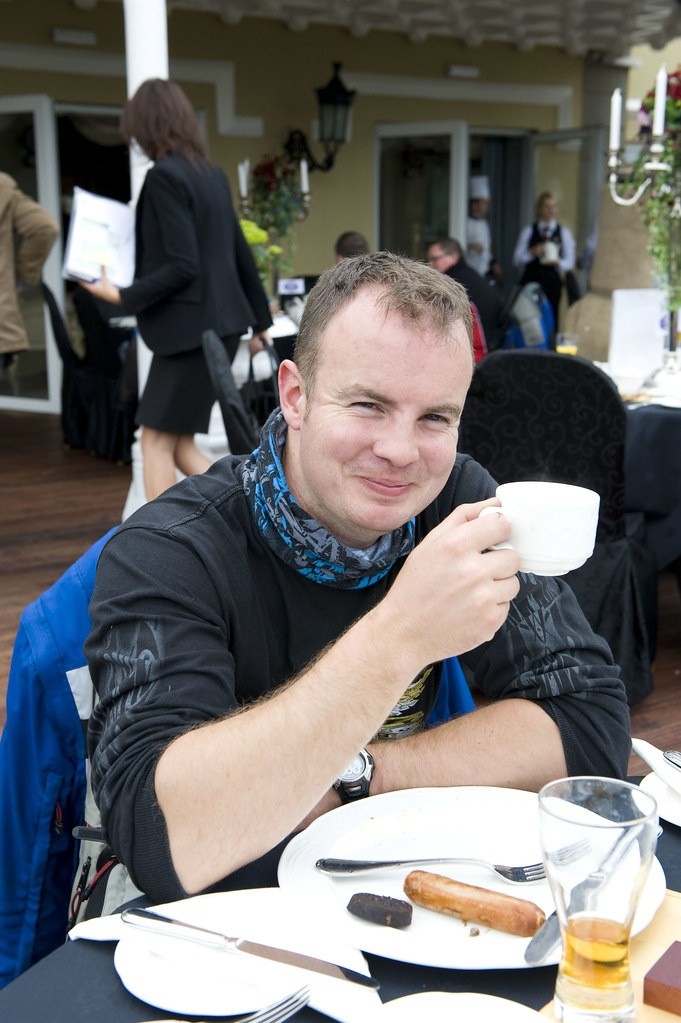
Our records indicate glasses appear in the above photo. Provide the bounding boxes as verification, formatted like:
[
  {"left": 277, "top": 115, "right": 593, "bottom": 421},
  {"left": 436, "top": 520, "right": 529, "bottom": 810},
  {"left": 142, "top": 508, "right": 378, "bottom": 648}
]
[{"left": 426, "top": 253, "right": 448, "bottom": 262}]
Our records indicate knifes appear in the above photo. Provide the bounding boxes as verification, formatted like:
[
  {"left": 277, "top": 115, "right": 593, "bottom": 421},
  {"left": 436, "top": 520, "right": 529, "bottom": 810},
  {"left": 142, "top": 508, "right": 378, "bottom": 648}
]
[
  {"left": 120, "top": 909, "right": 382, "bottom": 991},
  {"left": 313, "top": 833, "right": 595, "bottom": 884},
  {"left": 523, "top": 819, "right": 646, "bottom": 965}
]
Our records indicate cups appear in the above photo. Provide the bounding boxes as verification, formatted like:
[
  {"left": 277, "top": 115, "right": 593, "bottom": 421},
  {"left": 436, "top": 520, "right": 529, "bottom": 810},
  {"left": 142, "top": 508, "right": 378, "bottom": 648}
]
[
  {"left": 531, "top": 775, "right": 658, "bottom": 1023},
  {"left": 475, "top": 481, "right": 601, "bottom": 577}
]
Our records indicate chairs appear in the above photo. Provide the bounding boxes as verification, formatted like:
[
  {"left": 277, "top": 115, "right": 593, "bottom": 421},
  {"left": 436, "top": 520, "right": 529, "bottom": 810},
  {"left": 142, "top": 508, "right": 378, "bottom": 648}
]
[{"left": 199, "top": 326, "right": 659, "bottom": 707}]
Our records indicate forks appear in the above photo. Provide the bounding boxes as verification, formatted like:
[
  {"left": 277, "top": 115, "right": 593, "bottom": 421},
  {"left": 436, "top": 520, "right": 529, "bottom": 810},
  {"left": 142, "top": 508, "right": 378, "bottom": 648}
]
[{"left": 229, "top": 984, "right": 311, "bottom": 1023}]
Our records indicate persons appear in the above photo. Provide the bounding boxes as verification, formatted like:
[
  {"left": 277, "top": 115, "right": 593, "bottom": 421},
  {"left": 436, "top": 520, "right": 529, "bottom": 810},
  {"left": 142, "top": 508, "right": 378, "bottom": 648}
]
[
  {"left": 333, "top": 189, "right": 576, "bottom": 357},
  {"left": 88, "top": 250, "right": 632, "bottom": 906},
  {"left": 69, "top": 78, "right": 274, "bottom": 505},
  {"left": 0, "top": 171, "right": 57, "bottom": 375}
]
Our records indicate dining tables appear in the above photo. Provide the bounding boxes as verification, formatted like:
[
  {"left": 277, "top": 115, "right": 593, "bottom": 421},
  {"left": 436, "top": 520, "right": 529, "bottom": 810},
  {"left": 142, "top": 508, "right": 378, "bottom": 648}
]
[{"left": 1, "top": 776, "right": 681, "bottom": 1023}]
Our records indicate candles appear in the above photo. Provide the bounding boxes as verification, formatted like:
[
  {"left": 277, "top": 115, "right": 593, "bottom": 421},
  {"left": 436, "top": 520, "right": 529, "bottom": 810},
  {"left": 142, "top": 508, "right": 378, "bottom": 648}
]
[
  {"left": 652, "top": 63, "right": 669, "bottom": 135},
  {"left": 300, "top": 157, "right": 312, "bottom": 193},
  {"left": 608, "top": 87, "right": 623, "bottom": 152},
  {"left": 236, "top": 163, "right": 246, "bottom": 199}
]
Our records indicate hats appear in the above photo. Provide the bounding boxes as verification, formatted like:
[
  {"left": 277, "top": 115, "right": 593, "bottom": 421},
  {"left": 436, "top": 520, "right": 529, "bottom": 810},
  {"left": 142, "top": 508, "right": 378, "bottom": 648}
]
[{"left": 469, "top": 176, "right": 490, "bottom": 200}]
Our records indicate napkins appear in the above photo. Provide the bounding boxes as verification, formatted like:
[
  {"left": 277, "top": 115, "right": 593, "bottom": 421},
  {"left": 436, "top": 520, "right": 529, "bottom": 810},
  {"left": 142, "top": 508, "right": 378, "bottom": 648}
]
[{"left": 65, "top": 878, "right": 382, "bottom": 1023}]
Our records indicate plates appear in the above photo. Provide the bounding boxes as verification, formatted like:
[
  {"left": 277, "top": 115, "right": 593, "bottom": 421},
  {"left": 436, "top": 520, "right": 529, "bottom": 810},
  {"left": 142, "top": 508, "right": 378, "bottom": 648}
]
[
  {"left": 633, "top": 770, "right": 681, "bottom": 829},
  {"left": 277, "top": 784, "right": 666, "bottom": 969},
  {"left": 348, "top": 991, "right": 562, "bottom": 1022},
  {"left": 112, "top": 895, "right": 330, "bottom": 1018}
]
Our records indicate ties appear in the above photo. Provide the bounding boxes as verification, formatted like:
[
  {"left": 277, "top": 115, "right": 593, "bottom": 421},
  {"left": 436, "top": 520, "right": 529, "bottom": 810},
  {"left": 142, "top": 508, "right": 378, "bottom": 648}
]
[{"left": 542, "top": 226, "right": 548, "bottom": 237}]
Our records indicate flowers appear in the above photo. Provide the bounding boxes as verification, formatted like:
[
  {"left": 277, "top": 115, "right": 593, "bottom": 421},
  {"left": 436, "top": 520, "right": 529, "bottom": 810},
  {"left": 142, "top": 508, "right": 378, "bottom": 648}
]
[
  {"left": 626, "top": 66, "right": 681, "bottom": 313},
  {"left": 244, "top": 159, "right": 303, "bottom": 236}
]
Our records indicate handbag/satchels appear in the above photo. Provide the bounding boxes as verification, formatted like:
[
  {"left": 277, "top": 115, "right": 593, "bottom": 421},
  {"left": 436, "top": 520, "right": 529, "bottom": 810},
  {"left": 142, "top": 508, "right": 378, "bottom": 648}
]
[{"left": 237, "top": 338, "right": 281, "bottom": 427}]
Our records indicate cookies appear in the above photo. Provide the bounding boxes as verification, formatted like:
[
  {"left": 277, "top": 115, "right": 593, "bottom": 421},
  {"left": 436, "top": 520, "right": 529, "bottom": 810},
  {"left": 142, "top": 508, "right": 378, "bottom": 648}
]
[{"left": 346, "top": 892, "right": 413, "bottom": 927}]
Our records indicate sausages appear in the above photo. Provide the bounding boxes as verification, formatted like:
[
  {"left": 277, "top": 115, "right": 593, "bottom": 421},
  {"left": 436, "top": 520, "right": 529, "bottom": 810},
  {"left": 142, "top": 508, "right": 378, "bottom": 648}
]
[{"left": 405, "top": 871, "right": 547, "bottom": 938}]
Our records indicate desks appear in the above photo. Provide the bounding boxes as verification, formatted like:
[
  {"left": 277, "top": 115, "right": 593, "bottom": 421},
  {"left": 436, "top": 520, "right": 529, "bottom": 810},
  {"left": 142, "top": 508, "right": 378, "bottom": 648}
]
[{"left": 135, "top": 304, "right": 298, "bottom": 425}]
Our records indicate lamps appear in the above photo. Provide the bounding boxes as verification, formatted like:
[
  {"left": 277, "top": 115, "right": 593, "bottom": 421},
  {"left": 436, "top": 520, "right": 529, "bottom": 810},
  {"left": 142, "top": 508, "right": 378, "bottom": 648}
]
[{"left": 283, "top": 60, "right": 360, "bottom": 172}]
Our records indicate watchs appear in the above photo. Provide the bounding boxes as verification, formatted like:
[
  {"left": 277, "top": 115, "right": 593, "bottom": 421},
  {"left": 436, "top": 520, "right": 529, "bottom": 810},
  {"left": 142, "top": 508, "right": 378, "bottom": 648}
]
[{"left": 332, "top": 749, "right": 376, "bottom": 803}]
